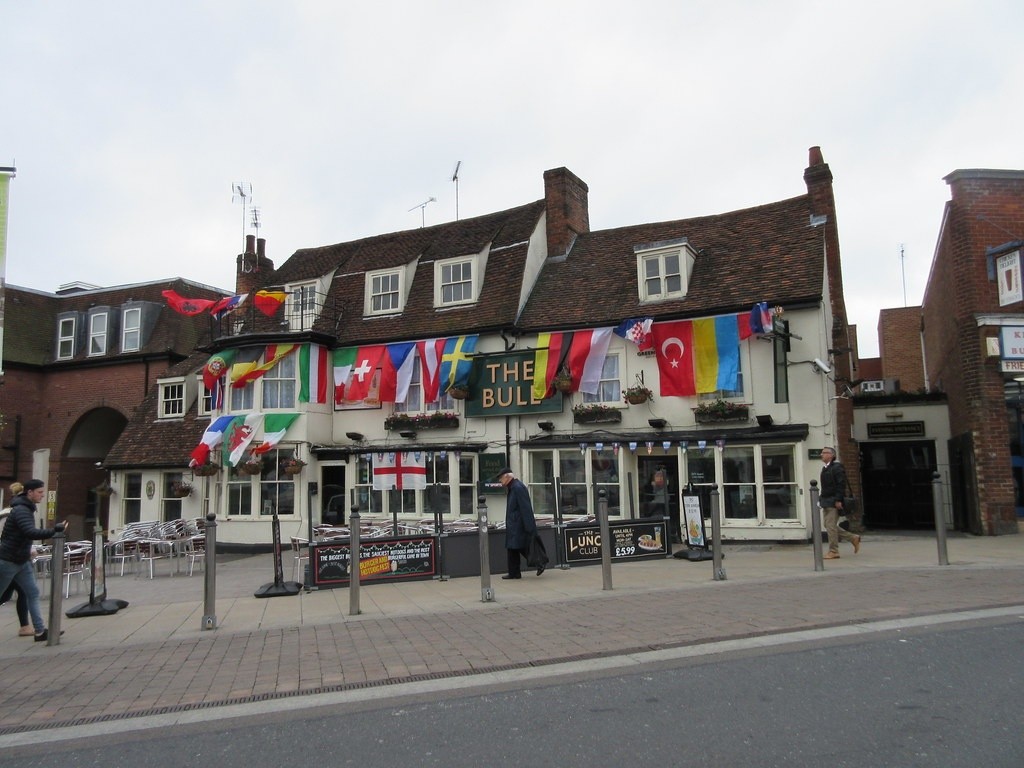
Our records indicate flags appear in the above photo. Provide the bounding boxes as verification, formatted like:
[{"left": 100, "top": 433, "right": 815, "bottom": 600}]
[
  {"left": 533, "top": 331, "right": 573, "bottom": 400},
  {"left": 232, "top": 342, "right": 299, "bottom": 389},
  {"left": 653, "top": 302, "right": 774, "bottom": 396},
  {"left": 188, "top": 415, "right": 235, "bottom": 464},
  {"left": 251, "top": 413, "right": 301, "bottom": 456},
  {"left": 614, "top": 316, "right": 653, "bottom": 350},
  {"left": 203, "top": 348, "right": 238, "bottom": 392},
  {"left": 223, "top": 415, "right": 264, "bottom": 466},
  {"left": 372, "top": 452, "right": 428, "bottom": 489},
  {"left": 416, "top": 338, "right": 447, "bottom": 403},
  {"left": 333, "top": 346, "right": 357, "bottom": 404},
  {"left": 162, "top": 290, "right": 250, "bottom": 320},
  {"left": 572, "top": 328, "right": 613, "bottom": 395},
  {"left": 298, "top": 344, "right": 327, "bottom": 403},
  {"left": 231, "top": 345, "right": 266, "bottom": 385},
  {"left": 438, "top": 334, "right": 479, "bottom": 397},
  {"left": 378, "top": 342, "right": 418, "bottom": 403},
  {"left": 346, "top": 344, "right": 386, "bottom": 400}
]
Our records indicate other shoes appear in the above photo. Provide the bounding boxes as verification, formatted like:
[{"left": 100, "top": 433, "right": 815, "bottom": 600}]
[
  {"left": 537, "top": 564, "right": 545, "bottom": 576},
  {"left": 823, "top": 552, "right": 840, "bottom": 559},
  {"left": 34, "top": 629, "right": 64, "bottom": 642},
  {"left": 502, "top": 573, "right": 521, "bottom": 579},
  {"left": 852, "top": 535, "right": 861, "bottom": 553},
  {"left": 19, "top": 625, "right": 35, "bottom": 636}
]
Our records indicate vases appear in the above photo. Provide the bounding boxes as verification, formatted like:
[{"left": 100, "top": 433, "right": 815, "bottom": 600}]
[
  {"left": 555, "top": 380, "right": 571, "bottom": 392},
  {"left": 194, "top": 468, "right": 217, "bottom": 476},
  {"left": 694, "top": 410, "right": 748, "bottom": 423},
  {"left": 574, "top": 411, "right": 621, "bottom": 423},
  {"left": 243, "top": 465, "right": 261, "bottom": 475},
  {"left": 629, "top": 394, "right": 647, "bottom": 404},
  {"left": 284, "top": 466, "right": 302, "bottom": 474},
  {"left": 450, "top": 389, "right": 468, "bottom": 400}
]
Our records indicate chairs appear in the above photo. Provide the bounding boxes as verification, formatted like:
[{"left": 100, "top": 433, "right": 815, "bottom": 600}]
[
  {"left": 290, "top": 514, "right": 597, "bottom": 583},
  {"left": 31, "top": 517, "right": 206, "bottom": 598}
]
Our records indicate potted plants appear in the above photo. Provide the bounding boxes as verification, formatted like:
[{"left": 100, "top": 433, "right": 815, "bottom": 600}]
[
  {"left": 91, "top": 480, "right": 117, "bottom": 496},
  {"left": 173, "top": 481, "right": 194, "bottom": 497}
]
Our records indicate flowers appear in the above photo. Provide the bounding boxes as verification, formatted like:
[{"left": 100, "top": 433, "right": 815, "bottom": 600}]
[
  {"left": 195, "top": 458, "right": 307, "bottom": 468},
  {"left": 551, "top": 375, "right": 749, "bottom": 411},
  {"left": 387, "top": 411, "right": 458, "bottom": 420}
]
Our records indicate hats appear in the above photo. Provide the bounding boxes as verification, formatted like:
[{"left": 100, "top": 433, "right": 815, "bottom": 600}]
[
  {"left": 23, "top": 479, "right": 44, "bottom": 494},
  {"left": 11, "top": 483, "right": 24, "bottom": 496},
  {"left": 496, "top": 468, "right": 511, "bottom": 478}
]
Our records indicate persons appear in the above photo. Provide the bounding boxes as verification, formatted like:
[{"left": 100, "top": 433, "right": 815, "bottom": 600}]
[
  {"left": 0, "top": 479, "right": 69, "bottom": 642},
  {"left": 817, "top": 447, "right": 860, "bottom": 559},
  {"left": 496, "top": 467, "right": 547, "bottom": 579}
]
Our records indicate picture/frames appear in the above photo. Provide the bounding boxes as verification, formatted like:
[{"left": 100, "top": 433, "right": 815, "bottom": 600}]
[{"left": 383, "top": 420, "right": 459, "bottom": 430}]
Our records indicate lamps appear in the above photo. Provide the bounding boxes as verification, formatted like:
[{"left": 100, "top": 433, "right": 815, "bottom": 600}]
[
  {"left": 648, "top": 419, "right": 666, "bottom": 428},
  {"left": 400, "top": 430, "right": 417, "bottom": 439},
  {"left": 346, "top": 432, "right": 363, "bottom": 441},
  {"left": 538, "top": 423, "right": 554, "bottom": 431},
  {"left": 755, "top": 414, "right": 774, "bottom": 427}
]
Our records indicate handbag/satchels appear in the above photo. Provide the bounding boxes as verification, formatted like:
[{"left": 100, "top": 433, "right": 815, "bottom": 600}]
[
  {"left": 838, "top": 498, "right": 858, "bottom": 516},
  {"left": 527, "top": 536, "right": 549, "bottom": 566}
]
